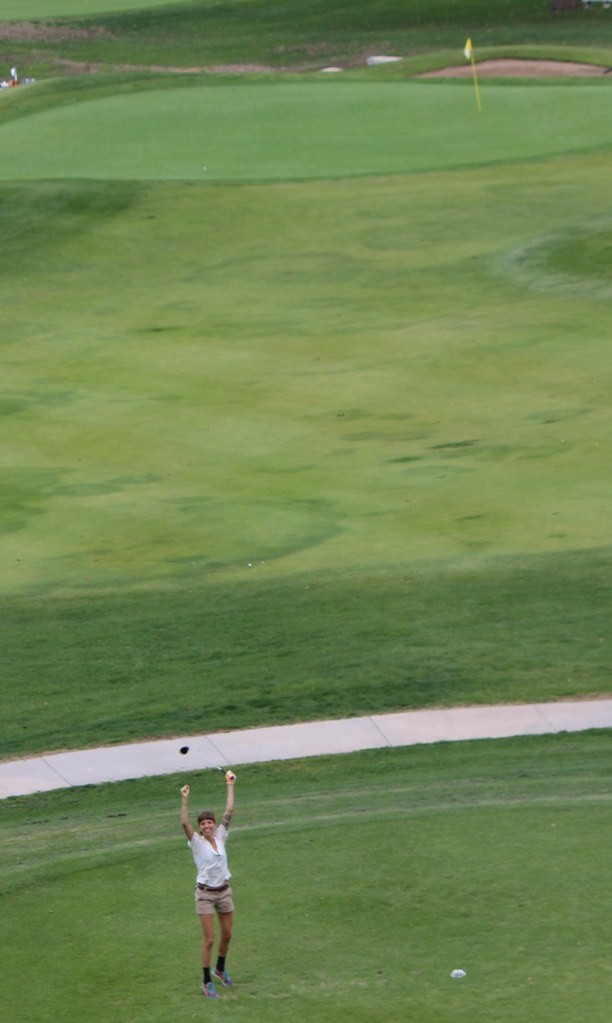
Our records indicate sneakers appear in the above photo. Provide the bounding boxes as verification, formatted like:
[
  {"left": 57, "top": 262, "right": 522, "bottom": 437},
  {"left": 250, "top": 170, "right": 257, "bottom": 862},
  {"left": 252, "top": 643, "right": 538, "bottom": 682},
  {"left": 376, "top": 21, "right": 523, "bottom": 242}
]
[
  {"left": 203, "top": 982, "right": 219, "bottom": 1000},
  {"left": 212, "top": 966, "right": 234, "bottom": 986}
]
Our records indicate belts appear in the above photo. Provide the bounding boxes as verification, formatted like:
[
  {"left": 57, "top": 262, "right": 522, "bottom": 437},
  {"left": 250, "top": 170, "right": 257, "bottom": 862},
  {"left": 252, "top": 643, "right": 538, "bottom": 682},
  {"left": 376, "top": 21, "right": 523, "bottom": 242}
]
[{"left": 198, "top": 884, "right": 228, "bottom": 891}]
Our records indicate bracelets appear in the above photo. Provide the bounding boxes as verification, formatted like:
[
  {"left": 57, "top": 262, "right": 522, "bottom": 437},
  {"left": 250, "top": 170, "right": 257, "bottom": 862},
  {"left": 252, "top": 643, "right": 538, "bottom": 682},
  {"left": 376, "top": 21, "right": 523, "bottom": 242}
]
[{"left": 226, "top": 781, "right": 235, "bottom": 785}]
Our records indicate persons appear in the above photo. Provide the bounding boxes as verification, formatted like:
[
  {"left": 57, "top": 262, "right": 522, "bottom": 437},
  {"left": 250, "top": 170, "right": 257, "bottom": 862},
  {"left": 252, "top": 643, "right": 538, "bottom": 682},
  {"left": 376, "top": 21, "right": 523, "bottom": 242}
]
[
  {"left": 181, "top": 768, "right": 237, "bottom": 996},
  {"left": 10, "top": 66, "right": 18, "bottom": 84}
]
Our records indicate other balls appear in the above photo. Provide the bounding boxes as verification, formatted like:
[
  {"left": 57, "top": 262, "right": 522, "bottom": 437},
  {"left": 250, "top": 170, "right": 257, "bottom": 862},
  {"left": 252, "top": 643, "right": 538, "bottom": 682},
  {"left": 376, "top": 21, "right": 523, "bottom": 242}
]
[{"left": 451, "top": 969, "right": 466, "bottom": 979}]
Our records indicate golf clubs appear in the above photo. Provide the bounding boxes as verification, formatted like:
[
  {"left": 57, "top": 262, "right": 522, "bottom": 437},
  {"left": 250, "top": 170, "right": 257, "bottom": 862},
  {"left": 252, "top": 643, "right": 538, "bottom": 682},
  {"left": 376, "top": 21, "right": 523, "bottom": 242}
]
[{"left": 180, "top": 746, "right": 234, "bottom": 780}]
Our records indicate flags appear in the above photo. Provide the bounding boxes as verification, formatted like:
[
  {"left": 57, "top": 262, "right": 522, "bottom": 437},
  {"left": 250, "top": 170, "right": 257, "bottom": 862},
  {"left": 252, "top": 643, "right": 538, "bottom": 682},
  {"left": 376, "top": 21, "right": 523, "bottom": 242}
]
[{"left": 465, "top": 39, "right": 472, "bottom": 61}]
